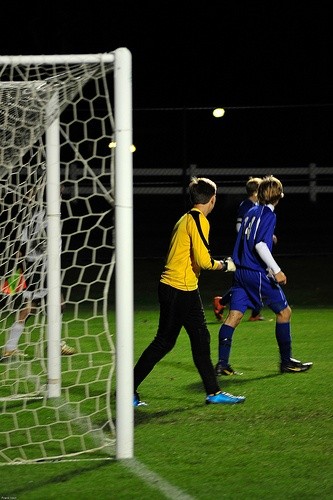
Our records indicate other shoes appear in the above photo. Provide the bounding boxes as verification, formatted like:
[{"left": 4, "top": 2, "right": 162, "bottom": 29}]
[
  {"left": 60, "top": 340, "right": 75, "bottom": 354},
  {"left": 3, "top": 349, "right": 27, "bottom": 358}
]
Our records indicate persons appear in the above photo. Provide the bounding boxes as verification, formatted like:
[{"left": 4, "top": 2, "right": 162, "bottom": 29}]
[
  {"left": 213, "top": 176, "right": 266, "bottom": 321},
  {"left": 215, "top": 175, "right": 313, "bottom": 376},
  {"left": 114, "top": 177, "right": 246, "bottom": 407},
  {"left": 0, "top": 182, "right": 75, "bottom": 358}
]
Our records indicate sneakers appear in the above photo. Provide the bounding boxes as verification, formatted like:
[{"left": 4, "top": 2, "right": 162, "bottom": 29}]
[
  {"left": 215, "top": 361, "right": 243, "bottom": 377},
  {"left": 280, "top": 358, "right": 313, "bottom": 373},
  {"left": 250, "top": 315, "right": 264, "bottom": 321},
  {"left": 113, "top": 389, "right": 140, "bottom": 406},
  {"left": 205, "top": 391, "right": 246, "bottom": 405},
  {"left": 213, "top": 296, "right": 226, "bottom": 321}
]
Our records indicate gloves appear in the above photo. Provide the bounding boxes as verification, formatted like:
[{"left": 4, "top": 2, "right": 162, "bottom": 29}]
[{"left": 221, "top": 257, "right": 236, "bottom": 272}]
